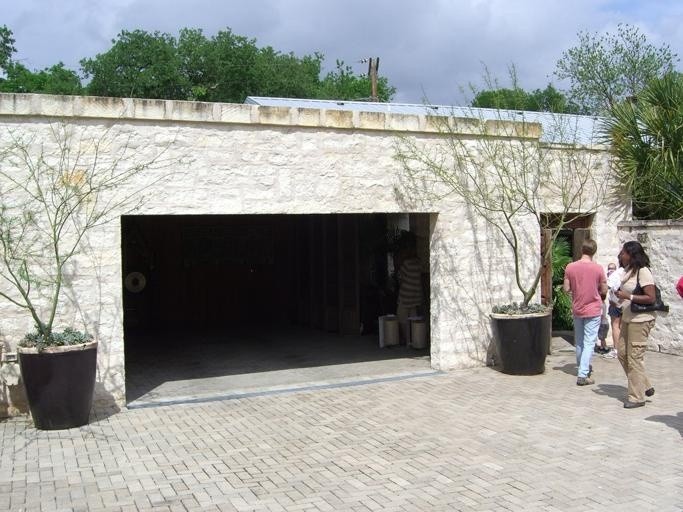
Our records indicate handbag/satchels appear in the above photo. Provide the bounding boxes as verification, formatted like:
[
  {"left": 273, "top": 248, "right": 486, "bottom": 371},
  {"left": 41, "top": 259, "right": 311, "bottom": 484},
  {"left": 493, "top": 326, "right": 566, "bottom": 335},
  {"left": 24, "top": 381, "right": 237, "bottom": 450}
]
[{"left": 632, "top": 267, "right": 669, "bottom": 311}]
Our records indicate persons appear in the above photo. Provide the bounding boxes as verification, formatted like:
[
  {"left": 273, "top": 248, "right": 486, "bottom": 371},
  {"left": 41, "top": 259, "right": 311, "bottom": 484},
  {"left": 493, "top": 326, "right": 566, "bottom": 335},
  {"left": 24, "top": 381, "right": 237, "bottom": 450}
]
[
  {"left": 393, "top": 246, "right": 422, "bottom": 345},
  {"left": 603, "top": 250, "right": 626, "bottom": 359},
  {"left": 563, "top": 239, "right": 607, "bottom": 386},
  {"left": 606, "top": 263, "right": 619, "bottom": 279},
  {"left": 616, "top": 241, "right": 656, "bottom": 408},
  {"left": 597, "top": 293, "right": 609, "bottom": 354}
]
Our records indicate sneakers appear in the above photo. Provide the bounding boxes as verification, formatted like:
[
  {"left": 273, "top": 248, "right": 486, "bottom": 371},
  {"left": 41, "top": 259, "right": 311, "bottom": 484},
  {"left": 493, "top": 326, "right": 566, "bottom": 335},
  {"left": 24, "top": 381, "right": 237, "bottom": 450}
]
[
  {"left": 601, "top": 343, "right": 619, "bottom": 359},
  {"left": 576, "top": 377, "right": 595, "bottom": 385},
  {"left": 587, "top": 365, "right": 593, "bottom": 375},
  {"left": 624, "top": 400, "right": 645, "bottom": 407},
  {"left": 646, "top": 388, "right": 654, "bottom": 397}
]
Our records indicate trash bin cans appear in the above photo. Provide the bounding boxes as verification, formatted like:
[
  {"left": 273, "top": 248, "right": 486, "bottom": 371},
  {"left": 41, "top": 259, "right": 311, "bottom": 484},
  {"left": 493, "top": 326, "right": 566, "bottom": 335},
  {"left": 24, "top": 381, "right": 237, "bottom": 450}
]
[
  {"left": 411, "top": 318, "right": 426, "bottom": 350},
  {"left": 383, "top": 317, "right": 399, "bottom": 349}
]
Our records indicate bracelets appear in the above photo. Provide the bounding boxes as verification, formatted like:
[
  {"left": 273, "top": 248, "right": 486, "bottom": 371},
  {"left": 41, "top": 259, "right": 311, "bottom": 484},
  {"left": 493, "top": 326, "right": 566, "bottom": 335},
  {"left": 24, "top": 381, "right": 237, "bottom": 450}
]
[{"left": 630, "top": 294, "right": 633, "bottom": 301}]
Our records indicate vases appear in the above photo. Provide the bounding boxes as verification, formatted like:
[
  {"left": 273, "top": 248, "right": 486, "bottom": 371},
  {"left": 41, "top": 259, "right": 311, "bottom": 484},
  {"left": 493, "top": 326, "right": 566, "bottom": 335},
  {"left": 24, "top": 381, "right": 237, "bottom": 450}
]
[
  {"left": 489, "top": 307, "right": 551, "bottom": 375},
  {"left": 17, "top": 340, "right": 98, "bottom": 429}
]
